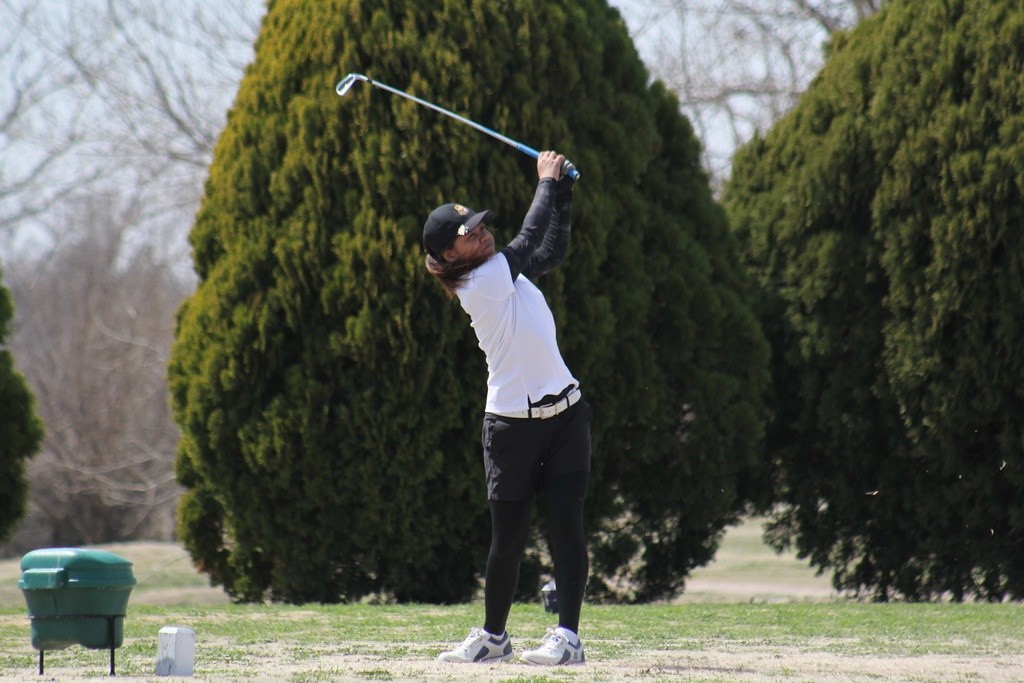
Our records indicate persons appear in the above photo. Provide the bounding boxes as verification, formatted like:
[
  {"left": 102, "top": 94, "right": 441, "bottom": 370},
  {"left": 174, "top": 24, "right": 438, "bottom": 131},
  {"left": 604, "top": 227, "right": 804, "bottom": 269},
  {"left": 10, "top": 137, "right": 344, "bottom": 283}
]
[{"left": 422, "top": 151, "right": 592, "bottom": 668}]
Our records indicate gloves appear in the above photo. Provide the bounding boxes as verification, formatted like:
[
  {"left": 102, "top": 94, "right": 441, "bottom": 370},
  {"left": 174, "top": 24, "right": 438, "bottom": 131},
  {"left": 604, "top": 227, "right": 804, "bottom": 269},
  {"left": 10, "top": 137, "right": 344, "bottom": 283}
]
[{"left": 556, "top": 159, "right": 577, "bottom": 196}]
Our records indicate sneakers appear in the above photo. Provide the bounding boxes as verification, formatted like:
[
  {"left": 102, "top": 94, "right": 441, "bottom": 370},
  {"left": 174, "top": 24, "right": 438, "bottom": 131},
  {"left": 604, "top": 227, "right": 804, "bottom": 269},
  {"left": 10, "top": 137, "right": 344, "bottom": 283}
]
[{"left": 439, "top": 627, "right": 585, "bottom": 666}]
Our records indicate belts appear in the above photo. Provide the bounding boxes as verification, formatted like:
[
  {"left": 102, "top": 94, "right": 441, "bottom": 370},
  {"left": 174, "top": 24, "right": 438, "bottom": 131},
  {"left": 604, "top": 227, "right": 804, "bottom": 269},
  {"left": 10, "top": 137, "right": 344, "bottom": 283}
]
[{"left": 493, "top": 386, "right": 581, "bottom": 420}]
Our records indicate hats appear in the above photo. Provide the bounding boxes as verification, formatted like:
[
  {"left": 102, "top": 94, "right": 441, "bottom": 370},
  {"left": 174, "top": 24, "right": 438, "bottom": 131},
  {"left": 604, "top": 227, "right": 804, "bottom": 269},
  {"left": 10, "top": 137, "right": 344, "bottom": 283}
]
[{"left": 422, "top": 203, "right": 490, "bottom": 261}]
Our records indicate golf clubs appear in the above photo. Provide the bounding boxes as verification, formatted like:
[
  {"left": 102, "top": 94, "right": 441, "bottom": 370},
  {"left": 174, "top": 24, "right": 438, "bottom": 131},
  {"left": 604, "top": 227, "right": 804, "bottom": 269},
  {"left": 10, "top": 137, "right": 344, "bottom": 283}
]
[{"left": 335, "top": 74, "right": 580, "bottom": 181}]
